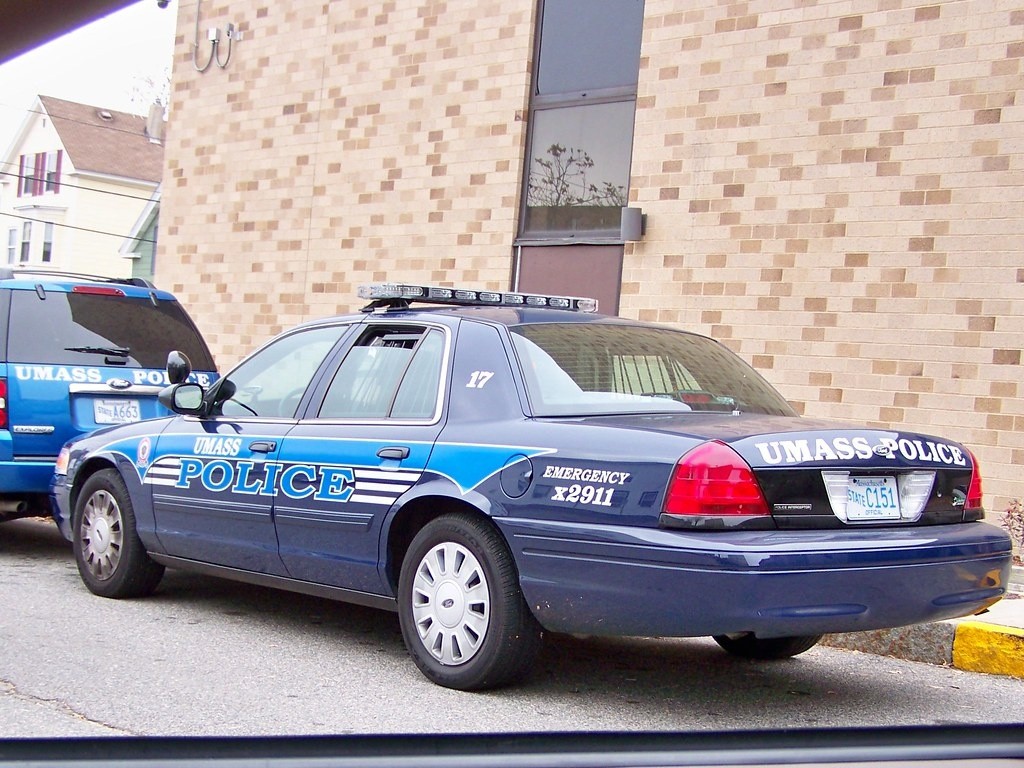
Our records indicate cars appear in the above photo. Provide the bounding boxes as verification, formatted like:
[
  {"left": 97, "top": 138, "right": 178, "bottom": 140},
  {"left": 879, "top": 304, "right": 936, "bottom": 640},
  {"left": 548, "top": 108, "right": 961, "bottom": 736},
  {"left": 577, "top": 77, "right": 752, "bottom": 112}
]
[
  {"left": 0, "top": 265, "right": 221, "bottom": 525},
  {"left": 47, "top": 283, "right": 1014, "bottom": 693}
]
